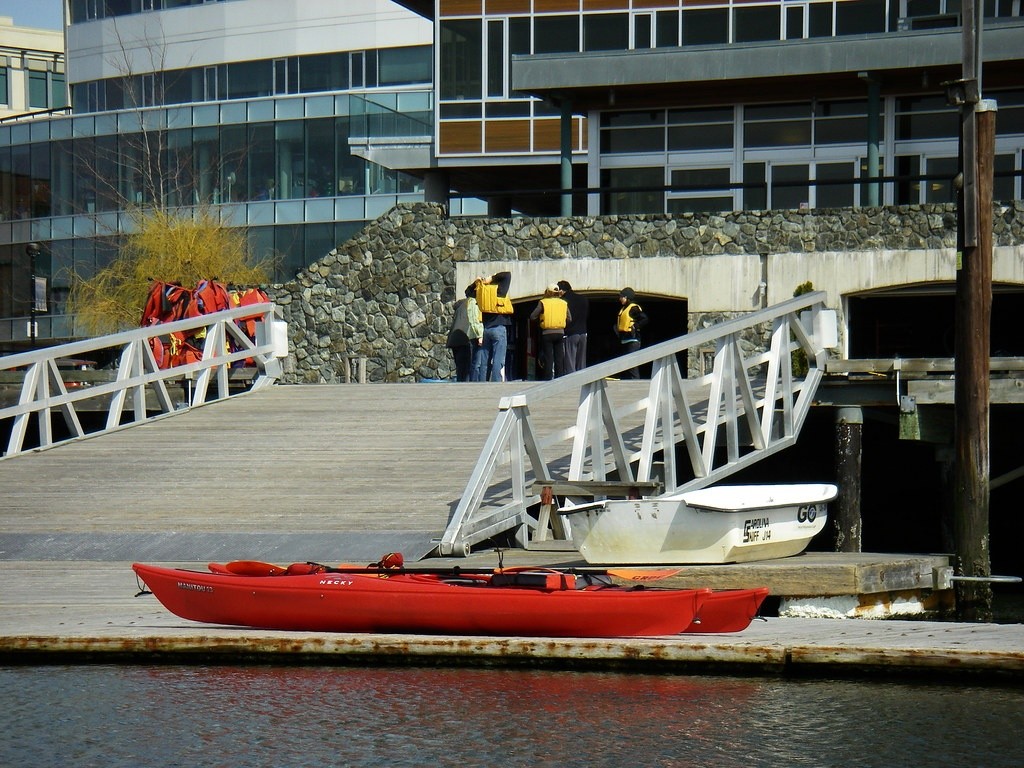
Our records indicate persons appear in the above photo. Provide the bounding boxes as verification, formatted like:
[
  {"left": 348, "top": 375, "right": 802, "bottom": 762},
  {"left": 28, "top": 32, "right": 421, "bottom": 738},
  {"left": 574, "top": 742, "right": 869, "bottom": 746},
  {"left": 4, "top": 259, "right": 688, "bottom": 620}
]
[
  {"left": 531, "top": 283, "right": 572, "bottom": 380},
  {"left": 557, "top": 281, "right": 587, "bottom": 375},
  {"left": 447, "top": 272, "right": 514, "bottom": 382},
  {"left": 614, "top": 287, "right": 647, "bottom": 379}
]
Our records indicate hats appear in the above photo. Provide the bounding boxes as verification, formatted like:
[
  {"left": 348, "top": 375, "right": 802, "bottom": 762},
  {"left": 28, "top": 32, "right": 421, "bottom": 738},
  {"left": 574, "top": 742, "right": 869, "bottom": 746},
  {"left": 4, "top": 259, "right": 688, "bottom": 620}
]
[
  {"left": 621, "top": 287, "right": 635, "bottom": 300},
  {"left": 546, "top": 284, "right": 559, "bottom": 293}
]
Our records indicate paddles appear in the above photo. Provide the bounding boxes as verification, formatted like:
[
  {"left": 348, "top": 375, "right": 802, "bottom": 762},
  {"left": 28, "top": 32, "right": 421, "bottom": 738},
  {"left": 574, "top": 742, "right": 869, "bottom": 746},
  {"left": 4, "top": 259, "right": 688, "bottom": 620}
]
[{"left": 302, "top": 564, "right": 686, "bottom": 583}]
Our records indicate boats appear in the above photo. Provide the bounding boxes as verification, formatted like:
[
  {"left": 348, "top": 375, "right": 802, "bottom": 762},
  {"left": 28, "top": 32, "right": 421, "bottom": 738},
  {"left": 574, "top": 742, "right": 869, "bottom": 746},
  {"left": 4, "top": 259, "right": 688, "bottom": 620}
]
[
  {"left": 551, "top": 480, "right": 839, "bottom": 570},
  {"left": 132, "top": 560, "right": 708, "bottom": 636},
  {"left": 206, "top": 559, "right": 770, "bottom": 634}
]
